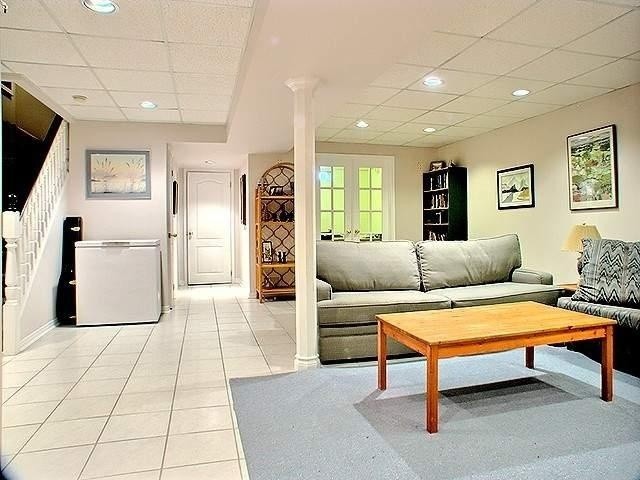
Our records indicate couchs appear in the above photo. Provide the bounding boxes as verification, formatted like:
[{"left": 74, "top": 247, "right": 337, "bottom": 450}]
[
  {"left": 315, "top": 234, "right": 560, "bottom": 365},
  {"left": 558, "top": 237, "right": 640, "bottom": 380}
]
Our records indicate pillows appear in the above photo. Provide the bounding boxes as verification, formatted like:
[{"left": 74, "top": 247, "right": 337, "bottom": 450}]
[
  {"left": 572, "top": 238, "right": 640, "bottom": 305},
  {"left": 416, "top": 234, "right": 522, "bottom": 292},
  {"left": 316, "top": 240, "right": 422, "bottom": 291}
]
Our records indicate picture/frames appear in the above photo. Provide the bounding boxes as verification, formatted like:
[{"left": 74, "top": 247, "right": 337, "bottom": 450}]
[
  {"left": 567, "top": 124, "right": 618, "bottom": 211},
  {"left": 497, "top": 164, "right": 535, "bottom": 210},
  {"left": 85, "top": 149, "right": 152, "bottom": 200}
]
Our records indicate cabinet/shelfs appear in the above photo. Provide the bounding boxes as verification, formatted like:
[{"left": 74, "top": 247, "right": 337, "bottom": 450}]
[
  {"left": 253, "top": 162, "right": 297, "bottom": 303},
  {"left": 423, "top": 168, "right": 467, "bottom": 241}
]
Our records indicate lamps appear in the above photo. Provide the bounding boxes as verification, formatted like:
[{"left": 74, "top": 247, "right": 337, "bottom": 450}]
[{"left": 558, "top": 223, "right": 602, "bottom": 259}]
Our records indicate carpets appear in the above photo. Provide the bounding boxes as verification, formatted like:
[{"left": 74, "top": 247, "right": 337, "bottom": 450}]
[{"left": 227, "top": 345, "right": 640, "bottom": 480}]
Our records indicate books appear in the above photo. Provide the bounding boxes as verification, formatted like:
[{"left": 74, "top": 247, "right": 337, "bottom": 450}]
[{"left": 423, "top": 172, "right": 449, "bottom": 242}]
